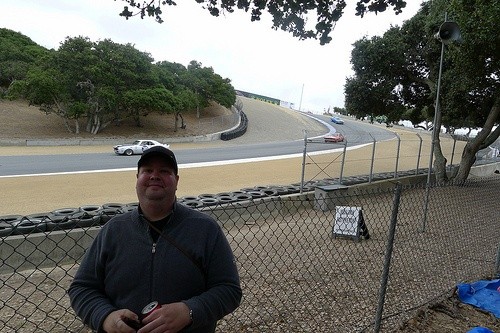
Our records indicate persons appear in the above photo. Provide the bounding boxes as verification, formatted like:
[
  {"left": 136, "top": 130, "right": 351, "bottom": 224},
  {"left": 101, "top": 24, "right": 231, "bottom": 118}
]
[{"left": 68, "top": 146, "right": 241, "bottom": 333}]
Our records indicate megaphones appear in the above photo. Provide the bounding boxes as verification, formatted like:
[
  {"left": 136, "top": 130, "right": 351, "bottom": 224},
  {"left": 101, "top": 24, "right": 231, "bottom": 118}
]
[{"left": 434, "top": 22, "right": 460, "bottom": 44}]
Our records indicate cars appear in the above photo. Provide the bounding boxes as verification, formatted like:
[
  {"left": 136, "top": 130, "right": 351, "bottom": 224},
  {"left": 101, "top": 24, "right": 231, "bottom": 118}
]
[
  {"left": 113, "top": 139, "right": 170, "bottom": 156},
  {"left": 330, "top": 117, "right": 344, "bottom": 124},
  {"left": 324, "top": 133, "right": 344, "bottom": 143}
]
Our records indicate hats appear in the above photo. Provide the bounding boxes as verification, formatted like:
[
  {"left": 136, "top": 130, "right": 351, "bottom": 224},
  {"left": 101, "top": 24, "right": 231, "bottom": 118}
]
[{"left": 137, "top": 146, "right": 178, "bottom": 175}]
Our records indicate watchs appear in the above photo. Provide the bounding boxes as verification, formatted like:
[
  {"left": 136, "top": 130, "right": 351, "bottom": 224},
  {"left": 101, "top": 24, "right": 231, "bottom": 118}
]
[{"left": 183, "top": 301, "right": 194, "bottom": 322}]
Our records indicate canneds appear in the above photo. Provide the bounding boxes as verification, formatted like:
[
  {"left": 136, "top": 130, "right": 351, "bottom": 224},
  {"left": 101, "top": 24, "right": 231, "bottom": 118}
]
[{"left": 142, "top": 302, "right": 161, "bottom": 318}]
